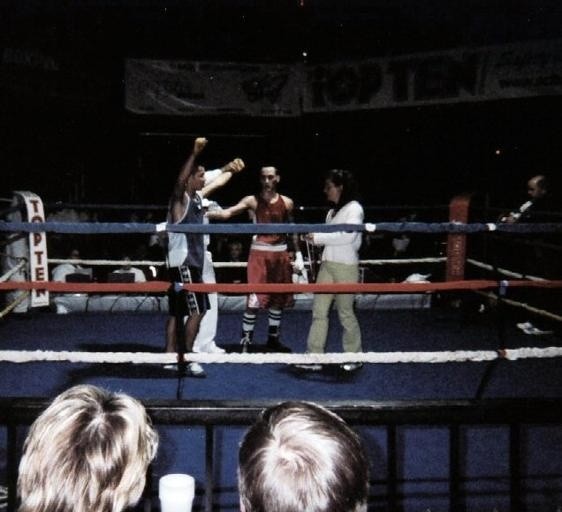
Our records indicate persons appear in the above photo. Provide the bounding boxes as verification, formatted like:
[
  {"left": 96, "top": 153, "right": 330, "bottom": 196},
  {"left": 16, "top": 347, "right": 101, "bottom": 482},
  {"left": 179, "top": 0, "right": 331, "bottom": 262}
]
[
  {"left": 235, "top": 395, "right": 374, "bottom": 510},
  {"left": 16, "top": 380, "right": 161, "bottom": 510},
  {"left": 166, "top": 137, "right": 246, "bottom": 383},
  {"left": 500, "top": 172, "right": 561, "bottom": 265},
  {"left": 47, "top": 202, "right": 169, "bottom": 283},
  {"left": 181, "top": 157, "right": 247, "bottom": 360},
  {"left": 292, "top": 169, "right": 370, "bottom": 376},
  {"left": 208, "top": 163, "right": 297, "bottom": 352},
  {"left": 205, "top": 209, "right": 424, "bottom": 290}
]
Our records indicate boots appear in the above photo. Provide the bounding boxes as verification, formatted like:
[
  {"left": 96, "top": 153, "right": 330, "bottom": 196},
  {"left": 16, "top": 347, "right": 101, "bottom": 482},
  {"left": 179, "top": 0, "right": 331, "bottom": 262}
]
[
  {"left": 239, "top": 329, "right": 253, "bottom": 353},
  {"left": 266, "top": 326, "right": 290, "bottom": 353}
]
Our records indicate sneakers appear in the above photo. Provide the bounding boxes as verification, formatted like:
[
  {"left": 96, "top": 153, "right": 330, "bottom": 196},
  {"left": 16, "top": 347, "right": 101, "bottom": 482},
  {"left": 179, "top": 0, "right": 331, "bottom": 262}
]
[
  {"left": 522, "top": 327, "right": 552, "bottom": 335},
  {"left": 187, "top": 361, "right": 206, "bottom": 379},
  {"left": 294, "top": 363, "right": 322, "bottom": 370},
  {"left": 341, "top": 362, "right": 364, "bottom": 371},
  {"left": 517, "top": 322, "right": 533, "bottom": 329}
]
